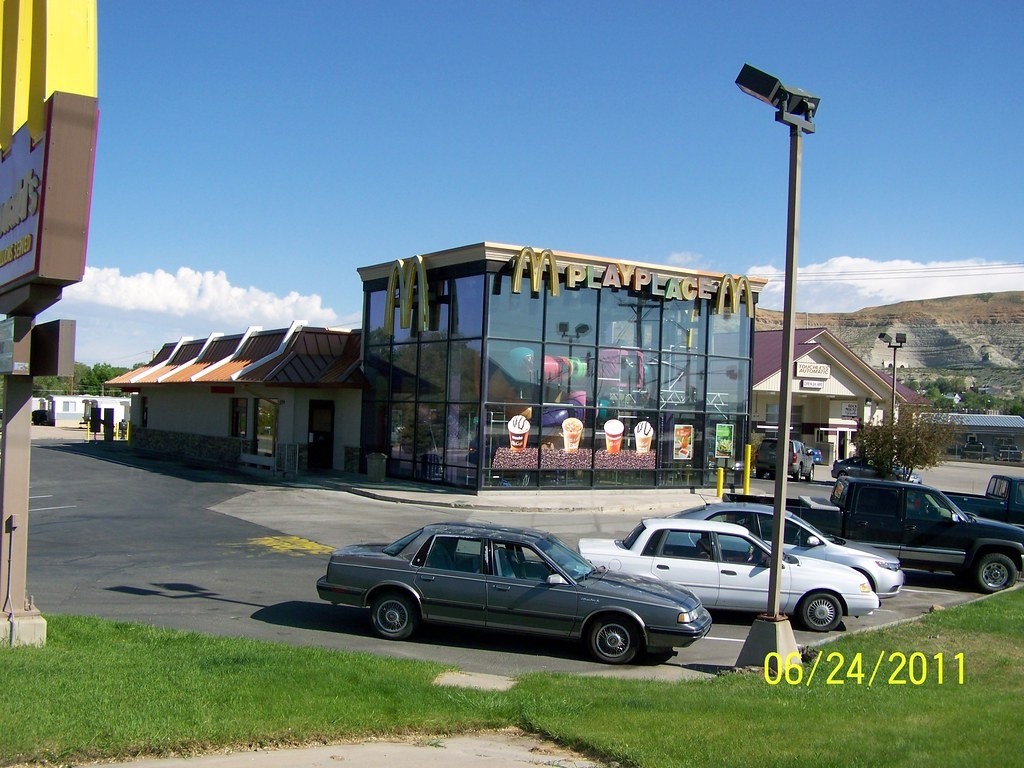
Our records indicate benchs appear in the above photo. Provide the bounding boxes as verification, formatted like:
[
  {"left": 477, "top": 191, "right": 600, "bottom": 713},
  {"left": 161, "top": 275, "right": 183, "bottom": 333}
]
[{"left": 428, "top": 543, "right": 453, "bottom": 569}]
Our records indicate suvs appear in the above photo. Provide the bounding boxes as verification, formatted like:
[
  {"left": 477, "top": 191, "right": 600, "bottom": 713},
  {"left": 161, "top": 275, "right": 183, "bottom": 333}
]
[{"left": 755, "top": 438, "right": 815, "bottom": 482}]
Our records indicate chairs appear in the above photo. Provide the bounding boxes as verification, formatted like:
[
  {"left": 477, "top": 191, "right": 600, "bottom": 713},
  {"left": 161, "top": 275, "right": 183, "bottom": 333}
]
[
  {"left": 495, "top": 546, "right": 516, "bottom": 578},
  {"left": 697, "top": 538, "right": 710, "bottom": 559}
]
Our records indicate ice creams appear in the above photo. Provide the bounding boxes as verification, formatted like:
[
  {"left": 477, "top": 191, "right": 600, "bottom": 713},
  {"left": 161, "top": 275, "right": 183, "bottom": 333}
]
[
  {"left": 634, "top": 421, "right": 653, "bottom": 437},
  {"left": 508, "top": 414, "right": 529, "bottom": 433}
]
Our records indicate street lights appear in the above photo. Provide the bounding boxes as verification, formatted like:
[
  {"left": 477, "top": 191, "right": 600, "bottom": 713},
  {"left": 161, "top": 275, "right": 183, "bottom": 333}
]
[
  {"left": 733, "top": 62, "right": 822, "bottom": 669},
  {"left": 878, "top": 333, "right": 907, "bottom": 420}
]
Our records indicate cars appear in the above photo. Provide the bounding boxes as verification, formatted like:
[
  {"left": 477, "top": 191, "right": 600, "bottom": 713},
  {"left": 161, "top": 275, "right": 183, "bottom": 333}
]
[
  {"left": 807, "top": 449, "right": 823, "bottom": 465},
  {"left": 465, "top": 433, "right": 542, "bottom": 486},
  {"left": 831, "top": 456, "right": 922, "bottom": 485},
  {"left": 317, "top": 521, "right": 712, "bottom": 666},
  {"left": 577, "top": 518, "right": 880, "bottom": 633},
  {"left": 642, "top": 502, "right": 906, "bottom": 600}
]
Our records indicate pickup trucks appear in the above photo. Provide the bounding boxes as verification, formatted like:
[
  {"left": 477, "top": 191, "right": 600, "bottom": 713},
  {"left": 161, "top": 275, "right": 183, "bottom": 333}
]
[
  {"left": 722, "top": 476, "right": 1024, "bottom": 593},
  {"left": 994, "top": 445, "right": 1023, "bottom": 462},
  {"left": 940, "top": 475, "right": 1024, "bottom": 523},
  {"left": 960, "top": 442, "right": 986, "bottom": 460}
]
